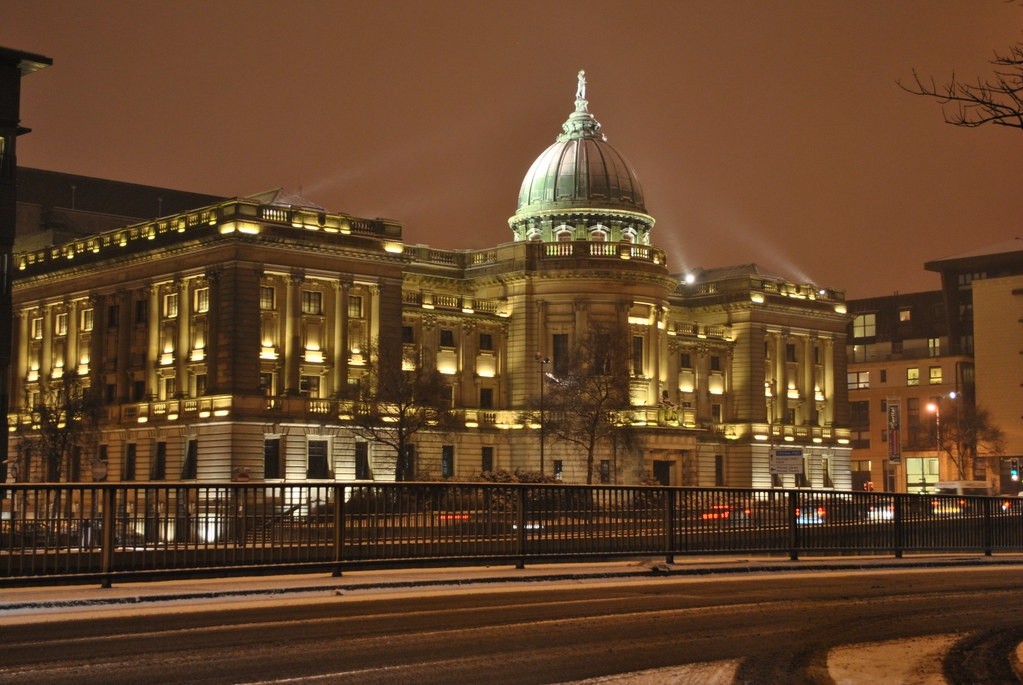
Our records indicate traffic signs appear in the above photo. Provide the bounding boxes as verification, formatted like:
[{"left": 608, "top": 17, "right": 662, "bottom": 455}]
[{"left": 768, "top": 448, "right": 802, "bottom": 474}]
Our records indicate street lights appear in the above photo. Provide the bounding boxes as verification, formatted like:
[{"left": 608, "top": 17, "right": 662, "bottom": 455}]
[
  {"left": 534, "top": 353, "right": 549, "bottom": 472},
  {"left": 926, "top": 405, "right": 940, "bottom": 456},
  {"left": 949, "top": 391, "right": 960, "bottom": 481}
]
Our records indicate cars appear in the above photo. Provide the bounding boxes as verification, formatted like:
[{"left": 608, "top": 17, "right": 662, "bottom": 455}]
[
  {"left": 704, "top": 500, "right": 785, "bottom": 525},
  {"left": 869, "top": 496, "right": 933, "bottom": 522},
  {"left": 793, "top": 497, "right": 867, "bottom": 524},
  {"left": 928, "top": 493, "right": 982, "bottom": 518},
  {"left": 991, "top": 494, "right": 1023, "bottom": 515}
]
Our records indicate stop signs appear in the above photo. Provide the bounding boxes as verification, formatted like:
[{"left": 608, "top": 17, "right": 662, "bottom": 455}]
[
  {"left": 90, "top": 463, "right": 106, "bottom": 480},
  {"left": 234, "top": 473, "right": 250, "bottom": 486}
]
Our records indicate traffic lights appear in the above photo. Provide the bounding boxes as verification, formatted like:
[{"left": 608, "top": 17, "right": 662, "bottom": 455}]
[
  {"left": 868, "top": 482, "right": 873, "bottom": 491},
  {"left": 1010, "top": 458, "right": 1019, "bottom": 481},
  {"left": 864, "top": 481, "right": 868, "bottom": 491}
]
